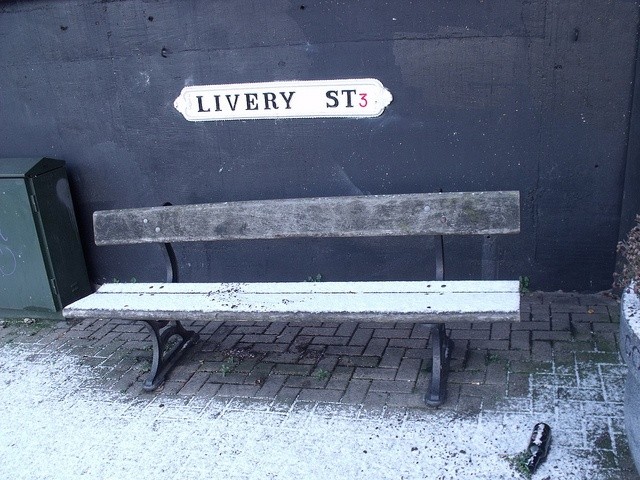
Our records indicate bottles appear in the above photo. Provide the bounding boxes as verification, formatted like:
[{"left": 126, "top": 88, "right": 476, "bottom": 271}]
[{"left": 523, "top": 421, "right": 553, "bottom": 474}]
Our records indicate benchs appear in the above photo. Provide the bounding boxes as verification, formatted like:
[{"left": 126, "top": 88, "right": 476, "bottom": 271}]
[{"left": 61, "top": 191, "right": 522, "bottom": 406}]
